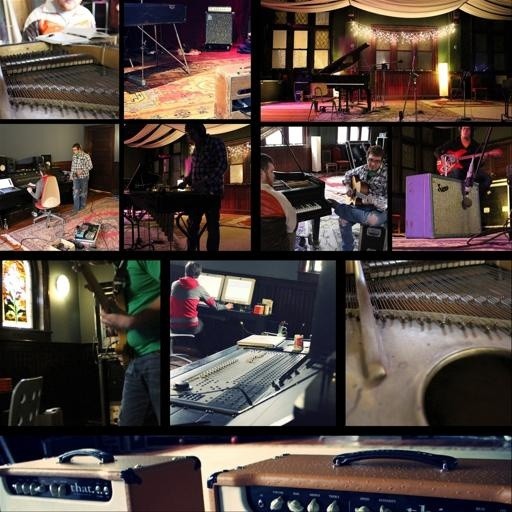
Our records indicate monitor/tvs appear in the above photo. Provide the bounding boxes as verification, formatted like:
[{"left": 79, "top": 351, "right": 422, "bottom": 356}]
[
  {"left": 196, "top": 273, "right": 225, "bottom": 301},
  {"left": 221, "top": 275, "right": 256, "bottom": 312}
]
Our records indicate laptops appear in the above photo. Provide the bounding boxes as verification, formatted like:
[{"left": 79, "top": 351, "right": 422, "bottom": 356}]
[{"left": 0, "top": 177, "right": 22, "bottom": 194}]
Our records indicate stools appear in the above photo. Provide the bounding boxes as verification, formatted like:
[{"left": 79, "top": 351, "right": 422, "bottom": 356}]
[
  {"left": 391, "top": 213, "right": 405, "bottom": 233},
  {"left": 33, "top": 208, "right": 67, "bottom": 227},
  {"left": 169, "top": 331, "right": 195, "bottom": 364}
]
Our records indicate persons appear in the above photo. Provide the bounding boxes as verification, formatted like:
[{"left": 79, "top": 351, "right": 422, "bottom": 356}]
[
  {"left": 69, "top": 142, "right": 94, "bottom": 212},
  {"left": 432, "top": 124, "right": 490, "bottom": 197},
  {"left": 23, "top": 0, "right": 97, "bottom": 43},
  {"left": 71, "top": 259, "right": 161, "bottom": 430},
  {"left": 178, "top": 123, "right": 228, "bottom": 251},
  {"left": 170, "top": 260, "right": 234, "bottom": 356},
  {"left": 27, "top": 162, "right": 62, "bottom": 218},
  {"left": 326, "top": 143, "right": 391, "bottom": 251},
  {"left": 260, "top": 151, "right": 297, "bottom": 235}
]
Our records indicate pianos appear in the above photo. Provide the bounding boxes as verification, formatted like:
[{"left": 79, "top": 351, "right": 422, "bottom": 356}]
[
  {"left": 260, "top": 125, "right": 332, "bottom": 244},
  {"left": 306, "top": 42, "right": 376, "bottom": 112}
]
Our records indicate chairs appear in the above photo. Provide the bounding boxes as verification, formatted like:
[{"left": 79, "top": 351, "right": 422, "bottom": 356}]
[
  {"left": 7, "top": 376, "right": 45, "bottom": 428},
  {"left": 335, "top": 147, "right": 349, "bottom": 172},
  {"left": 322, "top": 149, "right": 338, "bottom": 173},
  {"left": 307, "top": 87, "right": 340, "bottom": 118}
]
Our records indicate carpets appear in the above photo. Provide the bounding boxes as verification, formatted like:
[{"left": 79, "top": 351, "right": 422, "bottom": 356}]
[
  {"left": 123, "top": 60, "right": 217, "bottom": 95},
  {"left": 184, "top": 44, "right": 251, "bottom": 60},
  {"left": 2, "top": 197, "right": 119, "bottom": 251},
  {"left": 310, "top": 96, "right": 512, "bottom": 123}
]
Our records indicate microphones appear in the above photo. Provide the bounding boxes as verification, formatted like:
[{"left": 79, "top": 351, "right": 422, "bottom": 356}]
[
  {"left": 462, "top": 170, "right": 473, "bottom": 209},
  {"left": 395, "top": 60, "right": 403, "bottom": 63}
]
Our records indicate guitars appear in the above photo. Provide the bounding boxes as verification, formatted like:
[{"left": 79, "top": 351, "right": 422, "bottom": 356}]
[
  {"left": 436, "top": 149, "right": 501, "bottom": 175},
  {"left": 345, "top": 175, "right": 387, "bottom": 212},
  {"left": 73, "top": 262, "right": 134, "bottom": 372}
]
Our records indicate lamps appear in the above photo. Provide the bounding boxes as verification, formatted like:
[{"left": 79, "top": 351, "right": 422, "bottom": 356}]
[{"left": 437, "top": 62, "right": 450, "bottom": 101}]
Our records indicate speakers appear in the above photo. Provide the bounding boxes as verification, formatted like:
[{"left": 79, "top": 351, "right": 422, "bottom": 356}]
[
  {"left": 405, "top": 173, "right": 483, "bottom": 240},
  {"left": 205, "top": 13, "right": 233, "bottom": 52}
]
